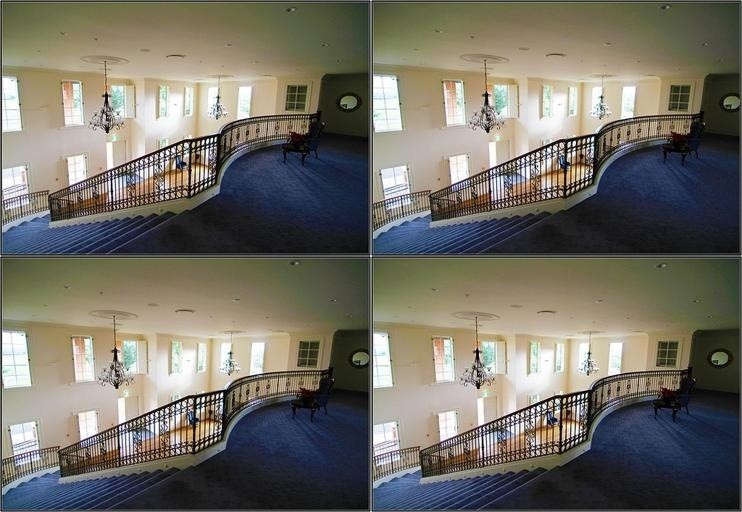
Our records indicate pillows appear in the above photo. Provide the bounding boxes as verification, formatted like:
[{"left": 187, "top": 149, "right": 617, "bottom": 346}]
[
  {"left": 300, "top": 386, "right": 311, "bottom": 400},
  {"left": 671, "top": 132, "right": 688, "bottom": 144},
  {"left": 290, "top": 132, "right": 308, "bottom": 145},
  {"left": 661, "top": 387, "right": 675, "bottom": 400}
]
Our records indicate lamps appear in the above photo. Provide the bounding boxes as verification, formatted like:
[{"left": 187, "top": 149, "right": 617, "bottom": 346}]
[
  {"left": 458, "top": 316, "right": 497, "bottom": 389},
  {"left": 590, "top": 76, "right": 611, "bottom": 120},
  {"left": 466, "top": 59, "right": 505, "bottom": 134},
  {"left": 219, "top": 332, "right": 240, "bottom": 376},
  {"left": 97, "top": 316, "right": 135, "bottom": 389},
  {"left": 87, "top": 60, "right": 126, "bottom": 134},
  {"left": 577, "top": 332, "right": 601, "bottom": 376},
  {"left": 207, "top": 77, "right": 229, "bottom": 120}
]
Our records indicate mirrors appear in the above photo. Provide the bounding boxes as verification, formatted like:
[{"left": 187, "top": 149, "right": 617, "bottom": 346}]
[
  {"left": 719, "top": 93, "right": 741, "bottom": 112},
  {"left": 336, "top": 93, "right": 361, "bottom": 112},
  {"left": 707, "top": 348, "right": 733, "bottom": 368},
  {"left": 348, "top": 348, "right": 369, "bottom": 368}
]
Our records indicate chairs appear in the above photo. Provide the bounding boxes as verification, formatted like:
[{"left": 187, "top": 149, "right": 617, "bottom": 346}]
[
  {"left": 560, "top": 155, "right": 570, "bottom": 170},
  {"left": 546, "top": 412, "right": 558, "bottom": 426},
  {"left": 176, "top": 156, "right": 187, "bottom": 170},
  {"left": 281, "top": 120, "right": 325, "bottom": 166},
  {"left": 291, "top": 377, "right": 336, "bottom": 421},
  {"left": 661, "top": 122, "right": 706, "bottom": 166},
  {"left": 653, "top": 376, "right": 696, "bottom": 421},
  {"left": 188, "top": 411, "right": 198, "bottom": 424}
]
